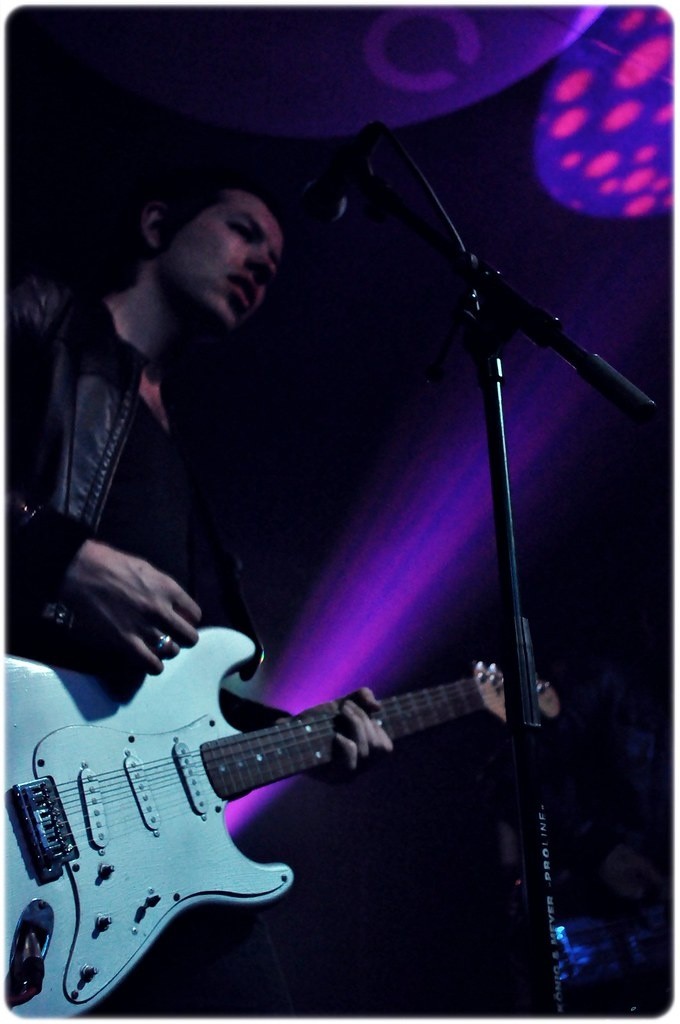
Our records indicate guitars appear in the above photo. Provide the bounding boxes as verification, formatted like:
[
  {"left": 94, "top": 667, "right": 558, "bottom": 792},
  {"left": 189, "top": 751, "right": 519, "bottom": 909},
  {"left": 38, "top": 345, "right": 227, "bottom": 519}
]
[{"left": 6, "top": 626, "right": 561, "bottom": 1018}]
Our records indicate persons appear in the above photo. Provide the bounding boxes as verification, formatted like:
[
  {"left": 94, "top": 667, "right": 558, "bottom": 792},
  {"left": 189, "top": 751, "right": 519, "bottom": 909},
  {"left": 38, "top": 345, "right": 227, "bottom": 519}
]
[{"left": 8, "top": 171, "right": 401, "bottom": 791}]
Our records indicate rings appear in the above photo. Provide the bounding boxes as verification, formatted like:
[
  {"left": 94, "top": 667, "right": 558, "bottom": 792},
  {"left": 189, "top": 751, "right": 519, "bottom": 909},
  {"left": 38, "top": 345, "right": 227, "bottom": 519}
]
[{"left": 153, "top": 632, "right": 171, "bottom": 656}]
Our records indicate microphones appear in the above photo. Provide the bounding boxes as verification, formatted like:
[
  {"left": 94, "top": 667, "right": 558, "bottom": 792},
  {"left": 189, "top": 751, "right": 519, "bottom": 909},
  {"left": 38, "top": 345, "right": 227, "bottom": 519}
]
[{"left": 303, "top": 119, "right": 384, "bottom": 221}]
